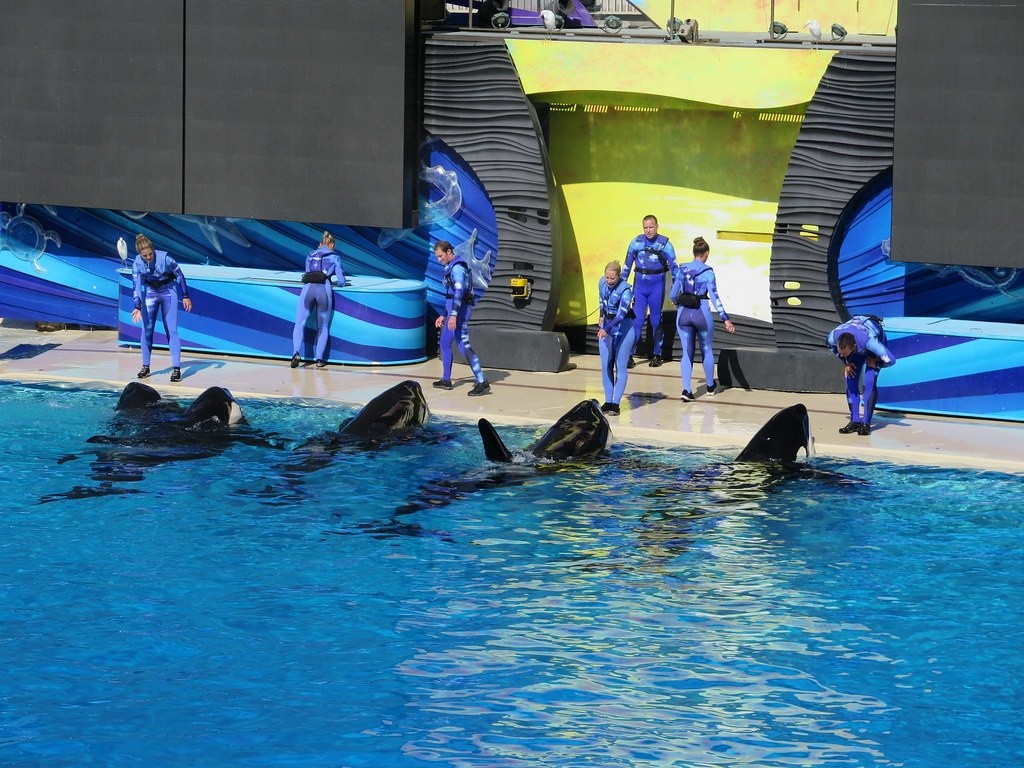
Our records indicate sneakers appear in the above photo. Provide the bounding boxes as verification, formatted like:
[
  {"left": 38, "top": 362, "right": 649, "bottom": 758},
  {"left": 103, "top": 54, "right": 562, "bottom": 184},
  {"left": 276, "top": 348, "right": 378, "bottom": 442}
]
[
  {"left": 468, "top": 379, "right": 489, "bottom": 396},
  {"left": 137, "top": 367, "right": 151, "bottom": 378},
  {"left": 707, "top": 380, "right": 717, "bottom": 396},
  {"left": 627, "top": 356, "right": 634, "bottom": 368},
  {"left": 170, "top": 369, "right": 182, "bottom": 381},
  {"left": 681, "top": 390, "right": 695, "bottom": 401},
  {"left": 858, "top": 423, "right": 871, "bottom": 435},
  {"left": 432, "top": 380, "right": 453, "bottom": 390},
  {"left": 840, "top": 422, "right": 860, "bottom": 433},
  {"left": 649, "top": 355, "right": 662, "bottom": 367}
]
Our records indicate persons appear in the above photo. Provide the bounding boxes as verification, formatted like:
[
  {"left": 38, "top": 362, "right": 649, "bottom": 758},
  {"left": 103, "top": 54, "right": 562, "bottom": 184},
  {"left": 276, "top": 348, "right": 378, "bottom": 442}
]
[
  {"left": 669, "top": 236, "right": 736, "bottom": 402},
  {"left": 595, "top": 259, "right": 638, "bottom": 416},
  {"left": 290, "top": 229, "right": 347, "bottom": 369},
  {"left": 434, "top": 242, "right": 491, "bottom": 398},
  {"left": 619, "top": 213, "right": 678, "bottom": 369},
  {"left": 822, "top": 315, "right": 896, "bottom": 436},
  {"left": 133, "top": 232, "right": 191, "bottom": 383}
]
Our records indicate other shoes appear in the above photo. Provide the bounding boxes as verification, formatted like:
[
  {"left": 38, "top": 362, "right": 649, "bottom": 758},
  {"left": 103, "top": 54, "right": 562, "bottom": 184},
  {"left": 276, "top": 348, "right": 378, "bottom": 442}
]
[
  {"left": 601, "top": 402, "right": 611, "bottom": 413},
  {"left": 291, "top": 355, "right": 300, "bottom": 368},
  {"left": 317, "top": 360, "right": 325, "bottom": 366},
  {"left": 610, "top": 404, "right": 620, "bottom": 415}
]
